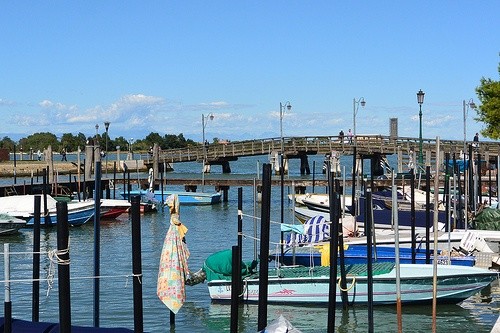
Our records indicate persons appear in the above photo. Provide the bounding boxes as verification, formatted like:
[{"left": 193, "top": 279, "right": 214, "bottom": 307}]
[
  {"left": 204, "top": 140, "right": 209, "bottom": 154},
  {"left": 474, "top": 132, "right": 478, "bottom": 153},
  {"left": 148, "top": 146, "right": 160, "bottom": 159},
  {"left": 100, "top": 148, "right": 106, "bottom": 164},
  {"left": 38, "top": 150, "right": 42, "bottom": 161},
  {"left": 347, "top": 128, "right": 352, "bottom": 144},
  {"left": 340, "top": 130, "right": 344, "bottom": 144},
  {"left": 61, "top": 148, "right": 67, "bottom": 161}
]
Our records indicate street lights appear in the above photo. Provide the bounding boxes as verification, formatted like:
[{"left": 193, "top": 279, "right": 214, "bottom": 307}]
[
  {"left": 414, "top": 88, "right": 426, "bottom": 179},
  {"left": 202, "top": 113, "right": 214, "bottom": 152},
  {"left": 462, "top": 98, "right": 477, "bottom": 159},
  {"left": 280, "top": 100, "right": 292, "bottom": 153},
  {"left": 103, "top": 121, "right": 111, "bottom": 178},
  {"left": 353, "top": 97, "right": 366, "bottom": 146},
  {"left": 95, "top": 122, "right": 100, "bottom": 141}
]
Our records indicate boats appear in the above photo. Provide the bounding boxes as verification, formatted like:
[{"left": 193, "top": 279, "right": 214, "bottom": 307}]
[
  {"left": 257, "top": 188, "right": 500, "bottom": 269},
  {"left": 120, "top": 190, "right": 221, "bottom": 205},
  {"left": 0, "top": 194, "right": 132, "bottom": 236},
  {"left": 185, "top": 249, "right": 500, "bottom": 306}
]
